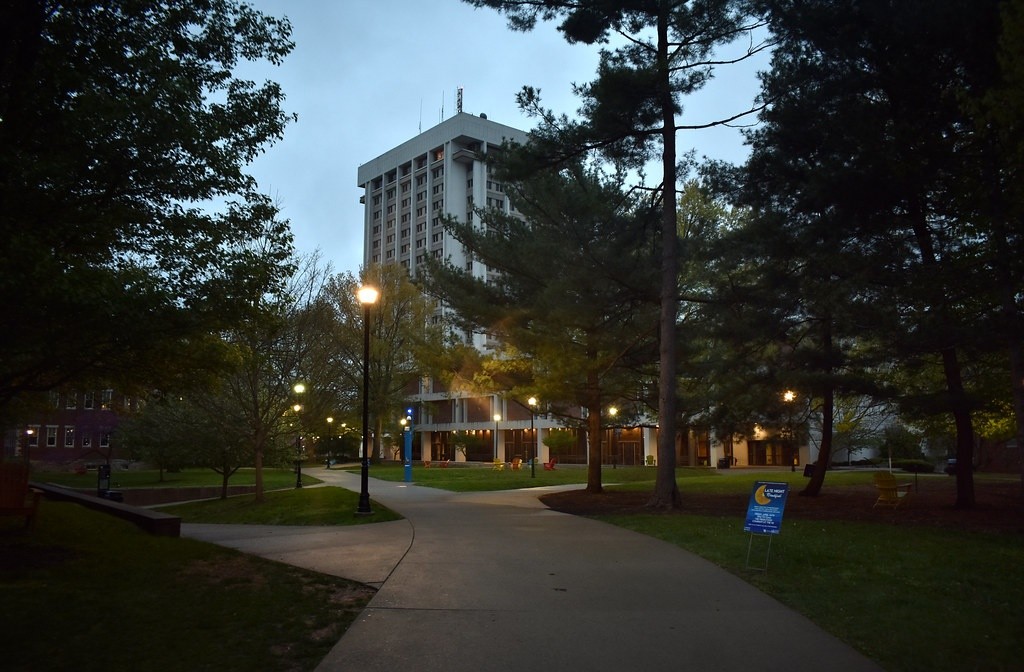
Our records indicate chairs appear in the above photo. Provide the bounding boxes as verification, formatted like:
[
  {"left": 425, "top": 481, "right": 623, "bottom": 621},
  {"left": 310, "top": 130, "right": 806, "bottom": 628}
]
[
  {"left": 493, "top": 459, "right": 505, "bottom": 470},
  {"left": 509, "top": 459, "right": 522, "bottom": 471},
  {"left": 543, "top": 458, "right": 557, "bottom": 471},
  {"left": 872, "top": 471, "right": 914, "bottom": 514},
  {"left": 423, "top": 459, "right": 432, "bottom": 468},
  {"left": 440, "top": 458, "right": 451, "bottom": 468}
]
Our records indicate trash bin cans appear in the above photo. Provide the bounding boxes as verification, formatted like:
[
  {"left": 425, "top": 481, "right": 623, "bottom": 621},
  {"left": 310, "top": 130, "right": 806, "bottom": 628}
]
[
  {"left": 103, "top": 489, "right": 124, "bottom": 503},
  {"left": 718, "top": 457, "right": 730, "bottom": 470},
  {"left": 647, "top": 455, "right": 654, "bottom": 465}
]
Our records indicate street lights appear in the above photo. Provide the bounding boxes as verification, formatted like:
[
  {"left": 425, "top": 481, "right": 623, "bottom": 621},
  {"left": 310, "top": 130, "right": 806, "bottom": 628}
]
[
  {"left": 609, "top": 407, "right": 618, "bottom": 469},
  {"left": 326, "top": 417, "right": 333, "bottom": 471},
  {"left": 399, "top": 418, "right": 407, "bottom": 464},
  {"left": 356, "top": 285, "right": 379, "bottom": 515},
  {"left": 341, "top": 423, "right": 346, "bottom": 463},
  {"left": 783, "top": 391, "right": 796, "bottom": 471},
  {"left": 526, "top": 397, "right": 536, "bottom": 478},
  {"left": 294, "top": 383, "right": 305, "bottom": 488}
]
